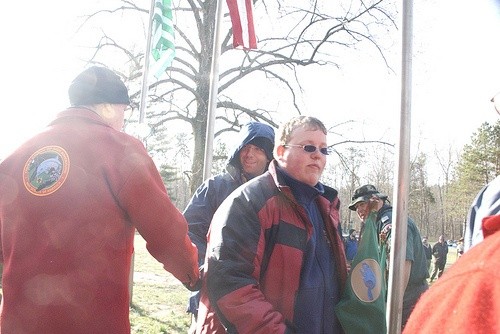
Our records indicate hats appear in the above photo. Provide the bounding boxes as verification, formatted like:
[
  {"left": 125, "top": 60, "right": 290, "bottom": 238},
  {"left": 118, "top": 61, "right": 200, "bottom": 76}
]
[
  {"left": 249, "top": 137, "right": 274, "bottom": 160},
  {"left": 68, "top": 66, "right": 129, "bottom": 104},
  {"left": 349, "top": 184, "right": 388, "bottom": 211},
  {"left": 349, "top": 229, "right": 356, "bottom": 235}
]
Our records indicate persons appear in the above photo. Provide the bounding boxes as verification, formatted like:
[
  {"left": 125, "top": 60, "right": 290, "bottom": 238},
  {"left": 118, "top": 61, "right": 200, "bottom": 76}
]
[
  {"left": 399, "top": 214, "right": 500, "bottom": 334},
  {"left": 195, "top": 115, "right": 346, "bottom": 334},
  {"left": 422, "top": 236, "right": 432, "bottom": 270},
  {"left": 346, "top": 184, "right": 429, "bottom": 334},
  {"left": 343, "top": 227, "right": 357, "bottom": 261},
  {"left": 179, "top": 121, "right": 275, "bottom": 322},
  {"left": 429, "top": 234, "right": 448, "bottom": 282},
  {"left": 463, "top": 176, "right": 500, "bottom": 255},
  {"left": 457, "top": 237, "right": 464, "bottom": 259},
  {"left": 0, "top": 65, "right": 198, "bottom": 333}
]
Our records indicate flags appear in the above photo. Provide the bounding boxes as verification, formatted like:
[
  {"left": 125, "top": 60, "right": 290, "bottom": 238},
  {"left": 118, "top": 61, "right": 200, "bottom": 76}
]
[
  {"left": 227, "top": 0, "right": 256, "bottom": 50},
  {"left": 151, "top": 0, "right": 174, "bottom": 79},
  {"left": 333, "top": 212, "right": 387, "bottom": 334}
]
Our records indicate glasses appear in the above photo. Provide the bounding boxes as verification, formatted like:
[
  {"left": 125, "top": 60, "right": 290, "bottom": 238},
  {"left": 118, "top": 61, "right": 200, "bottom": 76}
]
[
  {"left": 240, "top": 146, "right": 265, "bottom": 154},
  {"left": 284, "top": 144, "right": 332, "bottom": 155}
]
